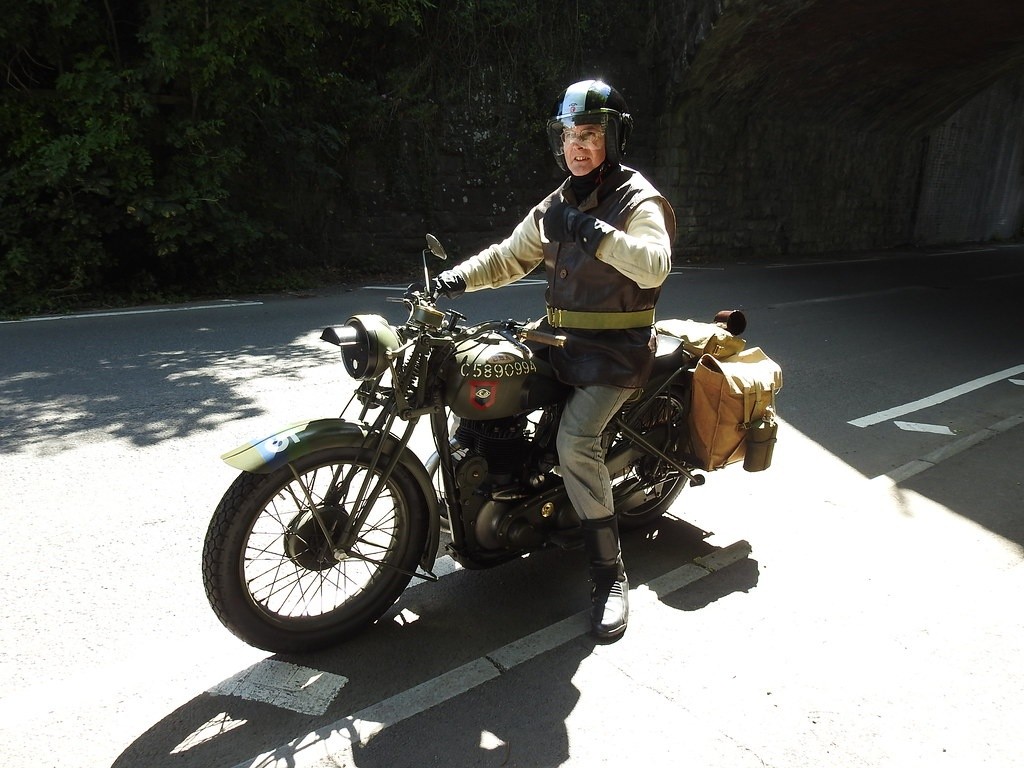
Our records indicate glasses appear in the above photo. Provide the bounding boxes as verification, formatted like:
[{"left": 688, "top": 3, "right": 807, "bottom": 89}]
[{"left": 558, "top": 129, "right": 602, "bottom": 144}]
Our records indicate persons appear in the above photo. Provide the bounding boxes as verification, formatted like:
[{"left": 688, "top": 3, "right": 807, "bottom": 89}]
[{"left": 402, "top": 78, "right": 675, "bottom": 645}]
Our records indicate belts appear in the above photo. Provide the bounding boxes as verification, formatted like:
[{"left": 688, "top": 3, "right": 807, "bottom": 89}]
[{"left": 545, "top": 306, "right": 656, "bottom": 329}]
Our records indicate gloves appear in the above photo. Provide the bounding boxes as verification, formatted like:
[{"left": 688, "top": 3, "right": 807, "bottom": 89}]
[
  {"left": 543, "top": 203, "right": 618, "bottom": 261},
  {"left": 402, "top": 269, "right": 467, "bottom": 308}
]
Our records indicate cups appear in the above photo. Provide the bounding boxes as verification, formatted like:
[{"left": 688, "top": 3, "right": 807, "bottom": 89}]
[{"left": 714, "top": 310, "right": 747, "bottom": 336}]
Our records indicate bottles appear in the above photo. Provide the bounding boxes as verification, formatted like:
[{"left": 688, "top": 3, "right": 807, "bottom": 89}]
[{"left": 744, "top": 407, "right": 778, "bottom": 472}]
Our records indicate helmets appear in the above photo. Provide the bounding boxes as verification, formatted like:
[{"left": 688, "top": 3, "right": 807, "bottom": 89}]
[{"left": 547, "top": 78, "right": 635, "bottom": 172}]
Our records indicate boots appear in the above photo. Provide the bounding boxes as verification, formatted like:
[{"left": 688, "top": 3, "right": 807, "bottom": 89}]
[{"left": 580, "top": 513, "right": 629, "bottom": 637}]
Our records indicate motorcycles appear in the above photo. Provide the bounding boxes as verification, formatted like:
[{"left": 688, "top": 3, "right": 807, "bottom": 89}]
[{"left": 203, "top": 232, "right": 748, "bottom": 653}]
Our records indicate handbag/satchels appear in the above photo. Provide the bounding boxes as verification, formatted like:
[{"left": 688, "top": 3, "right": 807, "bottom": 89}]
[
  {"left": 689, "top": 345, "right": 783, "bottom": 471},
  {"left": 654, "top": 318, "right": 746, "bottom": 362}
]
